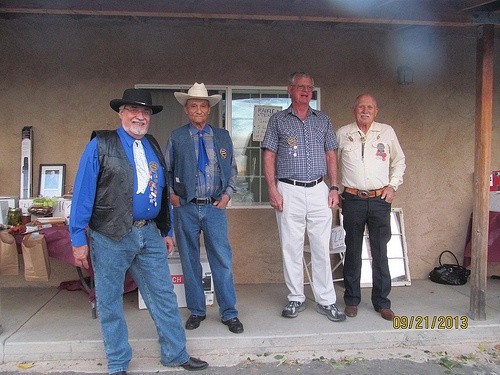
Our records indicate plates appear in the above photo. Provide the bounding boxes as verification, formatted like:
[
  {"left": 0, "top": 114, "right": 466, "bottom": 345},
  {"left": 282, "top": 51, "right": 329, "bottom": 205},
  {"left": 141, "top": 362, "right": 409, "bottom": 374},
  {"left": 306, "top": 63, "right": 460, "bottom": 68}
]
[{"left": 36, "top": 217, "right": 65, "bottom": 224}]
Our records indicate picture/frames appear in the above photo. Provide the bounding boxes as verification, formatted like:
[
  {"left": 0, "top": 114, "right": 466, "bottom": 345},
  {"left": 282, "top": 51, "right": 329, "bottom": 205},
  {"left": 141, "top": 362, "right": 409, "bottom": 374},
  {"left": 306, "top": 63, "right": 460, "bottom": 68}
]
[{"left": 38, "top": 163, "right": 65, "bottom": 198}]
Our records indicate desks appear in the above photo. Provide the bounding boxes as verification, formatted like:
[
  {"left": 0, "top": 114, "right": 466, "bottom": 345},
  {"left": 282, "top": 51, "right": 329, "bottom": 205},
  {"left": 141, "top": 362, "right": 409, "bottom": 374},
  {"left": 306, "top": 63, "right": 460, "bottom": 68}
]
[{"left": 9, "top": 224, "right": 138, "bottom": 320}]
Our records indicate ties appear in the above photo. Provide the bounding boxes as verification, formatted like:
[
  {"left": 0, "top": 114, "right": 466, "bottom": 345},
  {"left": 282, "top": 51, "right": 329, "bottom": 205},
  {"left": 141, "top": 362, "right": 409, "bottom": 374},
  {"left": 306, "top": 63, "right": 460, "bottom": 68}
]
[{"left": 197, "top": 130, "right": 210, "bottom": 176}]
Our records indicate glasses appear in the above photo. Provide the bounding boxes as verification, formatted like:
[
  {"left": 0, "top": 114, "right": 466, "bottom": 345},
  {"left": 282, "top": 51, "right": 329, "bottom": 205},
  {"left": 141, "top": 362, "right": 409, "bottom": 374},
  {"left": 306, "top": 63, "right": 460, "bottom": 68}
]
[
  {"left": 290, "top": 84, "right": 313, "bottom": 92},
  {"left": 124, "top": 107, "right": 152, "bottom": 116}
]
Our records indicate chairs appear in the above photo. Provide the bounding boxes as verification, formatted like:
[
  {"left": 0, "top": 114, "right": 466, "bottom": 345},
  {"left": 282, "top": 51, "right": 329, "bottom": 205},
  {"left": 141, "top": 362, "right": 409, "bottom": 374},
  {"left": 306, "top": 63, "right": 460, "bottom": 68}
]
[{"left": 303, "top": 246, "right": 346, "bottom": 303}]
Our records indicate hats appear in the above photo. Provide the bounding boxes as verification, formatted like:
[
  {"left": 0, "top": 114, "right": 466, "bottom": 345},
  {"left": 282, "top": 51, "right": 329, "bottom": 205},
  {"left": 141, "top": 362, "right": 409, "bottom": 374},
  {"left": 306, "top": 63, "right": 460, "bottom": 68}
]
[
  {"left": 174, "top": 81, "right": 222, "bottom": 107},
  {"left": 110, "top": 89, "right": 163, "bottom": 115}
]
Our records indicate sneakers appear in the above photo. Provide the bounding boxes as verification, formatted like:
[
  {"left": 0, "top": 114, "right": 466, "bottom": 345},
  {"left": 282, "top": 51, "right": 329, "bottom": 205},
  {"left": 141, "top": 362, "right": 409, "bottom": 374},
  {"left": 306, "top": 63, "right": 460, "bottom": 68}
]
[
  {"left": 315, "top": 303, "right": 347, "bottom": 321},
  {"left": 282, "top": 298, "right": 305, "bottom": 318}
]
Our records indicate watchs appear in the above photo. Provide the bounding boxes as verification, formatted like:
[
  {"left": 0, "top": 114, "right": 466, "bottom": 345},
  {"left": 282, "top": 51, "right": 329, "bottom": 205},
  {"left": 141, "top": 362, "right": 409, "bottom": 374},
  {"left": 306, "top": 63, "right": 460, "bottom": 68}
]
[{"left": 329, "top": 186, "right": 340, "bottom": 192}]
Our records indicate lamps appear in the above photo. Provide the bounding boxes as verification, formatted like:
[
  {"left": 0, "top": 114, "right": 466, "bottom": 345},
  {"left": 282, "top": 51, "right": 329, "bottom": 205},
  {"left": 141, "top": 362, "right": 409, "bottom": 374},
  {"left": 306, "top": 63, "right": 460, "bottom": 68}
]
[{"left": 398, "top": 64, "right": 414, "bottom": 85}]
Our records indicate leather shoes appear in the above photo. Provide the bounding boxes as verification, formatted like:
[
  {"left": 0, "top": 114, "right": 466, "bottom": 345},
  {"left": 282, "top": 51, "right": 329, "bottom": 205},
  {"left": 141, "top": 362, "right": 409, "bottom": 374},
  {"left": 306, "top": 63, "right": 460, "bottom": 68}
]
[
  {"left": 221, "top": 317, "right": 244, "bottom": 334},
  {"left": 185, "top": 314, "right": 206, "bottom": 330},
  {"left": 345, "top": 305, "right": 358, "bottom": 317},
  {"left": 374, "top": 308, "right": 395, "bottom": 321},
  {"left": 110, "top": 371, "right": 127, "bottom": 375},
  {"left": 183, "top": 357, "right": 208, "bottom": 371}
]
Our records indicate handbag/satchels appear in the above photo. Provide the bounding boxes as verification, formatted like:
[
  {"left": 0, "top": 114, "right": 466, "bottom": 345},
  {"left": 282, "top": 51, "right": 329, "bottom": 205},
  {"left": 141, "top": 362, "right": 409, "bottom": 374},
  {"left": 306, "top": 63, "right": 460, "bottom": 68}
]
[
  {"left": 0, "top": 232, "right": 18, "bottom": 275},
  {"left": 21, "top": 233, "right": 50, "bottom": 282},
  {"left": 429, "top": 250, "right": 471, "bottom": 286}
]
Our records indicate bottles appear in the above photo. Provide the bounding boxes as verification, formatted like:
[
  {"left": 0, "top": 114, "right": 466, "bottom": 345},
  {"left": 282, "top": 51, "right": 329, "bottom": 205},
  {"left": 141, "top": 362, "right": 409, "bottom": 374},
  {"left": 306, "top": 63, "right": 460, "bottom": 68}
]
[{"left": 7, "top": 208, "right": 22, "bottom": 226}]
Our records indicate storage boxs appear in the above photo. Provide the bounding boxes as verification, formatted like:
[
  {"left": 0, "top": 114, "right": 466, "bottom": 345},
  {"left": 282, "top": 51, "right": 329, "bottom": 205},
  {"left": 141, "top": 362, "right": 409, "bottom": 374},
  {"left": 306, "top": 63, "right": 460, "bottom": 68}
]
[
  {"left": 20, "top": 126, "right": 33, "bottom": 199},
  {"left": 138, "top": 259, "right": 216, "bottom": 310}
]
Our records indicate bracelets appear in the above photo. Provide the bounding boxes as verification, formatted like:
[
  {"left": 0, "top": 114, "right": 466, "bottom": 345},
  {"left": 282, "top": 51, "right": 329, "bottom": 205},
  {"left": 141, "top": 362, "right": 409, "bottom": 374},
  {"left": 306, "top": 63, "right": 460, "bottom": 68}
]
[{"left": 389, "top": 186, "right": 396, "bottom": 193}]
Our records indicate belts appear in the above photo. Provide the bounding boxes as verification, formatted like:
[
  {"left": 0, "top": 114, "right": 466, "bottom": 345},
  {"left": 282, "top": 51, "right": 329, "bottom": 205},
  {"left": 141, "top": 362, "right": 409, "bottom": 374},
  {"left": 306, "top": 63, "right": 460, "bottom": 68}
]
[
  {"left": 133, "top": 219, "right": 152, "bottom": 228},
  {"left": 344, "top": 187, "right": 384, "bottom": 199},
  {"left": 190, "top": 197, "right": 215, "bottom": 205},
  {"left": 278, "top": 177, "right": 324, "bottom": 187}
]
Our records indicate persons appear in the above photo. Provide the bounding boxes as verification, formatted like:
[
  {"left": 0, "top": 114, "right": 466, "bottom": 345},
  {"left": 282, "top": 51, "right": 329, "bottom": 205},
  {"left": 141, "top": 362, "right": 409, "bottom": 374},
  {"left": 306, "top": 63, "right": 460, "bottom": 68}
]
[
  {"left": 260, "top": 73, "right": 347, "bottom": 322},
  {"left": 45, "top": 170, "right": 59, "bottom": 189},
  {"left": 335, "top": 94, "right": 407, "bottom": 320},
  {"left": 69, "top": 89, "right": 209, "bottom": 375},
  {"left": 164, "top": 82, "right": 244, "bottom": 334}
]
